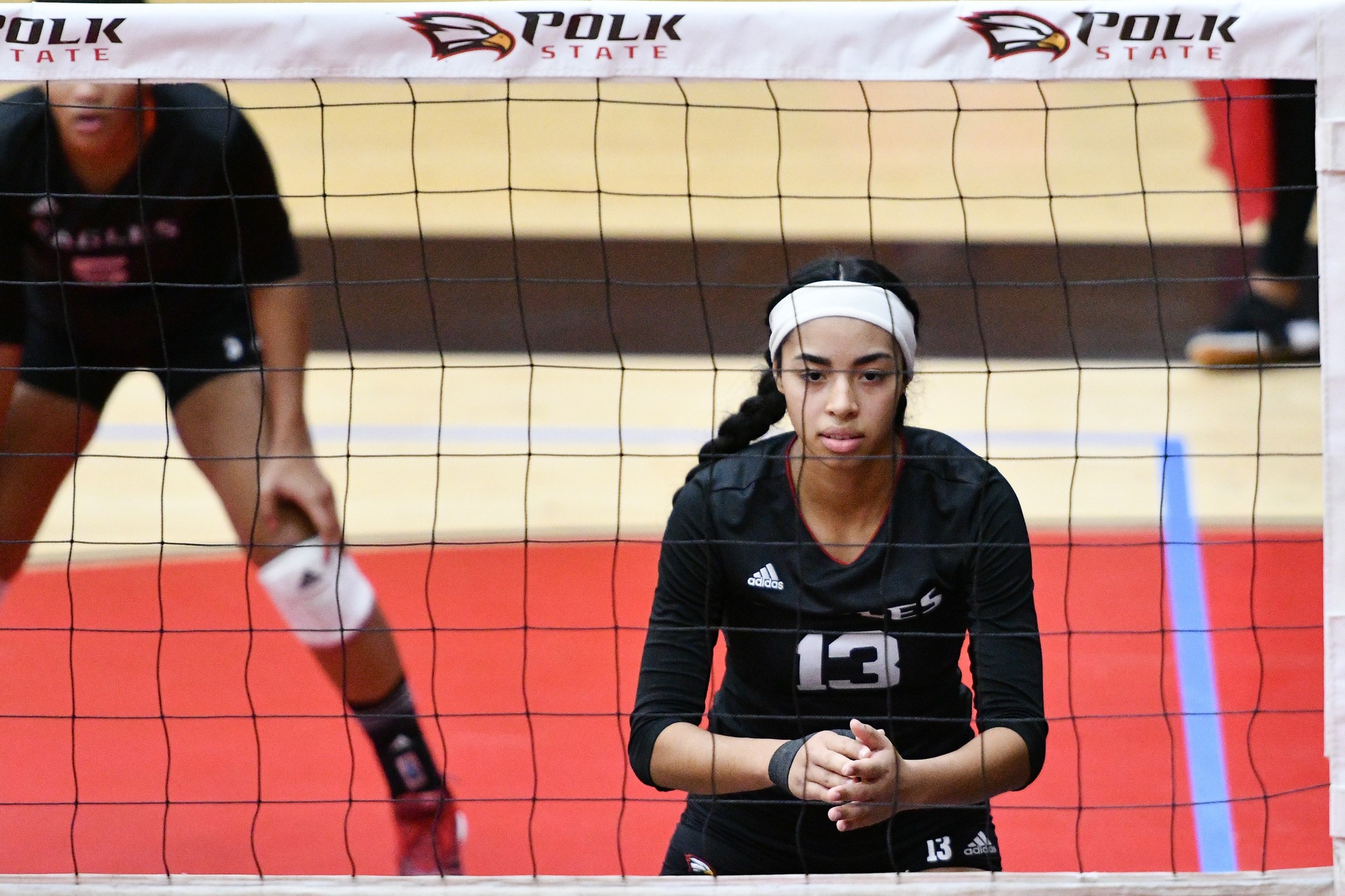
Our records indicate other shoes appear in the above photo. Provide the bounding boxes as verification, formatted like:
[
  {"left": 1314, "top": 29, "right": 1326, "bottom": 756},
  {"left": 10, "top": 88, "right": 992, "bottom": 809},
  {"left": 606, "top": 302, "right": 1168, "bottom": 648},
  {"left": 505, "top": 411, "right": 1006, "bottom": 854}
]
[
  {"left": 1184, "top": 288, "right": 1319, "bottom": 363},
  {"left": 390, "top": 788, "right": 468, "bottom": 877}
]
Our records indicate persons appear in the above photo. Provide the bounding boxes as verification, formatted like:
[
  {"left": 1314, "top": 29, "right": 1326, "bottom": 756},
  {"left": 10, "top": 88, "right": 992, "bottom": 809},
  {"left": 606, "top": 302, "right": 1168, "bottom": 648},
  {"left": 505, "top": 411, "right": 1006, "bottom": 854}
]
[
  {"left": 627, "top": 256, "right": 1049, "bottom": 874},
  {"left": 0, "top": 80, "right": 465, "bottom": 875},
  {"left": 1181, "top": 79, "right": 1320, "bottom": 369}
]
